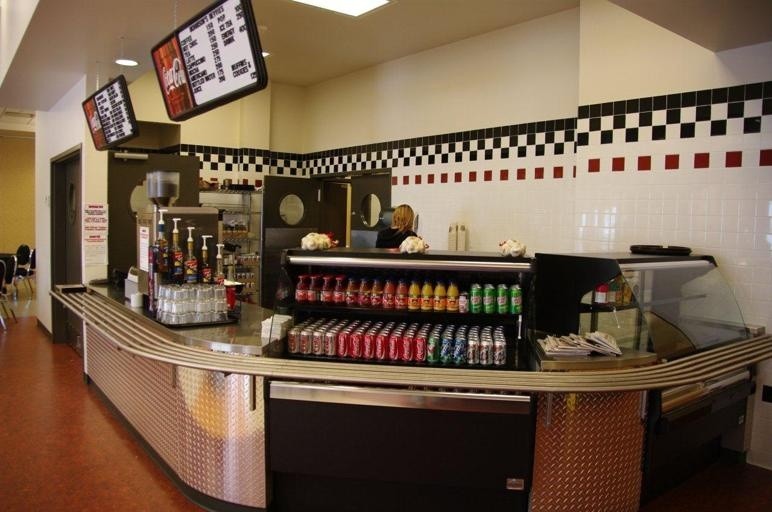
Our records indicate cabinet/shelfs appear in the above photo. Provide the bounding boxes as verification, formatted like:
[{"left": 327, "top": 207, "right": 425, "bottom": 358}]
[{"left": 224, "top": 210, "right": 261, "bottom": 303}]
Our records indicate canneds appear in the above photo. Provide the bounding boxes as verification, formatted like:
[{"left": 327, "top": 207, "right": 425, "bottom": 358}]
[
  {"left": 459, "top": 293, "right": 469, "bottom": 314},
  {"left": 469, "top": 283, "right": 523, "bottom": 315},
  {"left": 288, "top": 317, "right": 508, "bottom": 366}
]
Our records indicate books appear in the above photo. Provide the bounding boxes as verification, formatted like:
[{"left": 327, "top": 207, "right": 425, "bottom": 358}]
[{"left": 537, "top": 330, "right": 621, "bottom": 359}]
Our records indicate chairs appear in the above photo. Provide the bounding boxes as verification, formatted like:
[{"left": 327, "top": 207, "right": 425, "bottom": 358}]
[{"left": 1, "top": 244, "right": 36, "bottom": 332}]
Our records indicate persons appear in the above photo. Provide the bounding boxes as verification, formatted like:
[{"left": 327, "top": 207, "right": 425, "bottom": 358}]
[{"left": 376, "top": 203, "right": 426, "bottom": 247}]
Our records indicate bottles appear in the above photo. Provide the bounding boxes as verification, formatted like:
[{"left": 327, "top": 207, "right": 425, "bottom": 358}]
[
  {"left": 594, "top": 274, "right": 632, "bottom": 307},
  {"left": 294, "top": 273, "right": 458, "bottom": 311}
]
